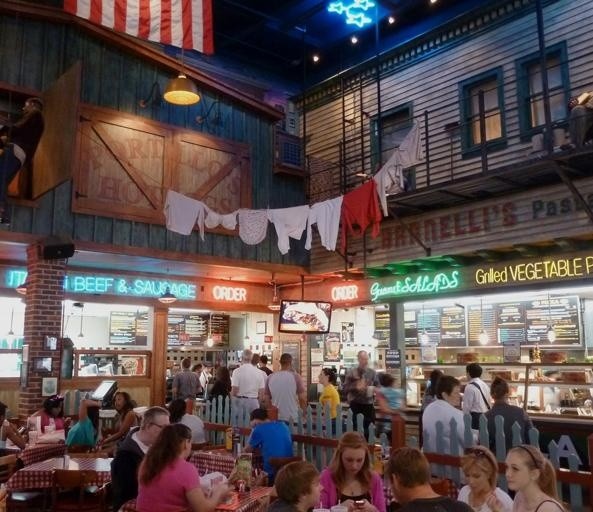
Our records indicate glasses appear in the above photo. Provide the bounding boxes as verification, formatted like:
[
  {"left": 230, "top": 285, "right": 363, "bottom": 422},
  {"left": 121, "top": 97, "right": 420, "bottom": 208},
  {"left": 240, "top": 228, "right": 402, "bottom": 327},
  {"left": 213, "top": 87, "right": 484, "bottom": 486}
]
[{"left": 463, "top": 447, "right": 495, "bottom": 468}]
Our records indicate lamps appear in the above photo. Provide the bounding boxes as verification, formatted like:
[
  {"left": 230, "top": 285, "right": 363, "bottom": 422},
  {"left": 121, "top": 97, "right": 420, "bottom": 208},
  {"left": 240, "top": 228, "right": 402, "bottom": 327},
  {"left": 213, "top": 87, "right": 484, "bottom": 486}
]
[
  {"left": 163, "top": 49, "right": 203, "bottom": 106},
  {"left": 205, "top": 311, "right": 212, "bottom": 349},
  {"left": 8, "top": 301, "right": 16, "bottom": 339},
  {"left": 476, "top": 294, "right": 488, "bottom": 347},
  {"left": 240, "top": 311, "right": 250, "bottom": 342},
  {"left": 78, "top": 304, "right": 84, "bottom": 339},
  {"left": 544, "top": 288, "right": 557, "bottom": 344},
  {"left": 418, "top": 298, "right": 431, "bottom": 346}
]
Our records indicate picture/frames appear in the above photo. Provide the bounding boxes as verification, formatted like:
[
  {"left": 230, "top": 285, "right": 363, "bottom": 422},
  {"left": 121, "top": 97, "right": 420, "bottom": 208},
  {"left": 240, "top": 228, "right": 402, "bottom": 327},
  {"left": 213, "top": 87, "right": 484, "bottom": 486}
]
[
  {"left": 42, "top": 333, "right": 59, "bottom": 352},
  {"left": 30, "top": 357, "right": 53, "bottom": 374}
]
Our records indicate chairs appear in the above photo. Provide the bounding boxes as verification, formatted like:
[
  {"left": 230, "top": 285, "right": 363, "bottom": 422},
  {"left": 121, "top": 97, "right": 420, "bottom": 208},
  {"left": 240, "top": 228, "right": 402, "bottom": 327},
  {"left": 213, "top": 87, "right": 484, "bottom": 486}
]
[{"left": 268, "top": 455, "right": 302, "bottom": 478}]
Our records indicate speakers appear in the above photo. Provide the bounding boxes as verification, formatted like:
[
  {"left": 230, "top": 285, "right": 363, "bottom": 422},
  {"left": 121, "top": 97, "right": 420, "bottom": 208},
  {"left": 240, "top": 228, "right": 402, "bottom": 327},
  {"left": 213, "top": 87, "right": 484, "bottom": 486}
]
[{"left": 45, "top": 243, "right": 75, "bottom": 259}]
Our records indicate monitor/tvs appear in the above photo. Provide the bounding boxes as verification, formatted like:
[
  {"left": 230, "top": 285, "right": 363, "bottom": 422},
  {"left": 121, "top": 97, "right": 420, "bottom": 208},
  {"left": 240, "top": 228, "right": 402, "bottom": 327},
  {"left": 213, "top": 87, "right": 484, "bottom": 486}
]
[
  {"left": 92, "top": 380, "right": 118, "bottom": 402},
  {"left": 276, "top": 300, "right": 332, "bottom": 334}
]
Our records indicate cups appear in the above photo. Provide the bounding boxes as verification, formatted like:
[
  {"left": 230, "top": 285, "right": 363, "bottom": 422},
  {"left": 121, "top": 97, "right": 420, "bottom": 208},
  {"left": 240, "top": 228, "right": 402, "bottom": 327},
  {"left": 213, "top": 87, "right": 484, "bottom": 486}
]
[
  {"left": 584, "top": 369, "right": 592, "bottom": 385},
  {"left": 437, "top": 355, "right": 443, "bottom": 363},
  {"left": 233, "top": 443, "right": 241, "bottom": 457},
  {"left": 28, "top": 431, "right": 36, "bottom": 446}
]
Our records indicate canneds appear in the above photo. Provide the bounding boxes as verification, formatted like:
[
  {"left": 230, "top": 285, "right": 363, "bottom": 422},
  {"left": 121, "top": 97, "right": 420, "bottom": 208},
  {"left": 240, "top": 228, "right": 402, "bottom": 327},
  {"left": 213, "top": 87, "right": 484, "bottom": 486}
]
[{"left": 237, "top": 480, "right": 246, "bottom": 493}]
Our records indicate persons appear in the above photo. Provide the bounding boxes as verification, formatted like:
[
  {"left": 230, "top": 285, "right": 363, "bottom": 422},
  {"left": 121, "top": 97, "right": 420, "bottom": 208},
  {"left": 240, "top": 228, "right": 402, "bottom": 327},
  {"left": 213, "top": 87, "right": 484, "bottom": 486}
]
[
  {"left": 566, "top": 98, "right": 592, "bottom": 148},
  {"left": 0, "top": 98, "right": 44, "bottom": 226}
]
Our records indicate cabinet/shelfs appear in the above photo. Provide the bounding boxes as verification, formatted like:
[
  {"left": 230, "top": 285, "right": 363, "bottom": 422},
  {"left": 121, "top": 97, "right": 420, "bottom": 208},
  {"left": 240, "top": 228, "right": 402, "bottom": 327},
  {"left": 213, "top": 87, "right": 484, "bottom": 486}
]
[
  {"left": 521, "top": 361, "right": 592, "bottom": 421},
  {"left": 405, "top": 360, "right": 528, "bottom": 413}
]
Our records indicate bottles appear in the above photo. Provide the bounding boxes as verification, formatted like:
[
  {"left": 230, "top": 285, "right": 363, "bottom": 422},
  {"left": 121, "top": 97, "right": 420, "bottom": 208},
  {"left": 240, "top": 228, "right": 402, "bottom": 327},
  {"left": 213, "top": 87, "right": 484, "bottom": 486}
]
[
  {"left": 63, "top": 455, "right": 69, "bottom": 466},
  {"left": 534, "top": 343, "right": 540, "bottom": 361},
  {"left": 236, "top": 460, "right": 251, "bottom": 491},
  {"left": 48, "top": 418, "right": 55, "bottom": 434},
  {"left": 541, "top": 368, "right": 547, "bottom": 376}
]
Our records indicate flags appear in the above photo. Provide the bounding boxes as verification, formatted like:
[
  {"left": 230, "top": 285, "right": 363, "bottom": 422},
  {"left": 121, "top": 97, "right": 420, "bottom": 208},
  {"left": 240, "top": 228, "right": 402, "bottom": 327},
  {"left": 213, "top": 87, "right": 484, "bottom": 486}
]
[{"left": 64, "top": 0, "right": 213, "bottom": 56}]
